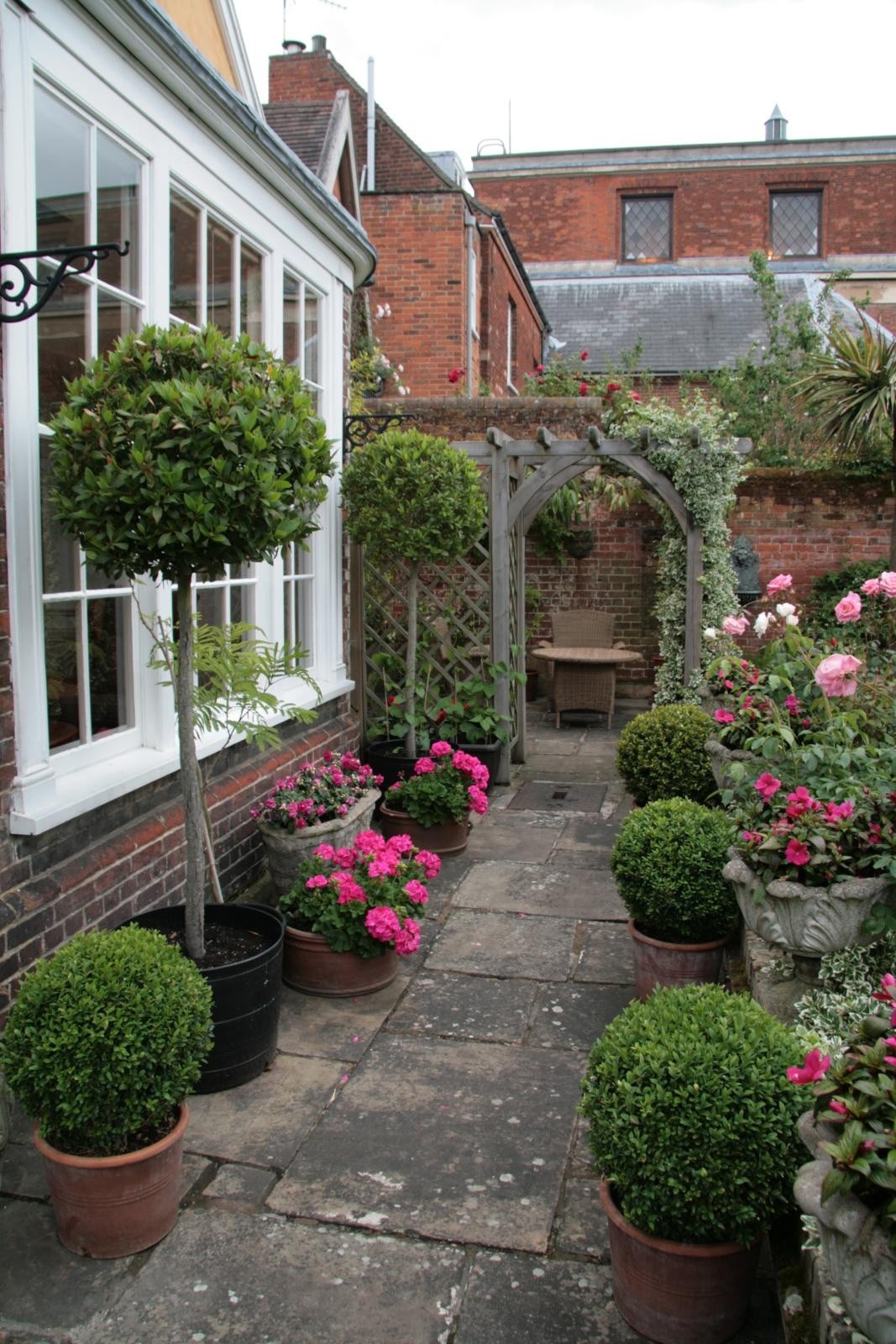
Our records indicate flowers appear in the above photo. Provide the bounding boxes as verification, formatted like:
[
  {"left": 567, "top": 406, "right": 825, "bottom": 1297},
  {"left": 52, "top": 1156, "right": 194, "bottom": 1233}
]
[
  {"left": 250, "top": 751, "right": 383, "bottom": 835},
  {"left": 704, "top": 572, "right": 895, "bottom": 1251},
  {"left": 278, "top": 830, "right": 442, "bottom": 958},
  {"left": 384, "top": 741, "right": 490, "bottom": 830}
]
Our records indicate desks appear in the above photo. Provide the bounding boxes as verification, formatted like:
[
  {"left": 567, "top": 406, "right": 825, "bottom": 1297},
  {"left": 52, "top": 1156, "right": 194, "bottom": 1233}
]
[{"left": 532, "top": 647, "right": 642, "bottom": 729}]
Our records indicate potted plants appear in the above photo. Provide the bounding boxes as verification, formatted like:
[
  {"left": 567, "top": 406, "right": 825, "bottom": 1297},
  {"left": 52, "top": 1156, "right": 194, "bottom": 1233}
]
[
  {"left": 0, "top": 921, "right": 216, "bottom": 1259},
  {"left": 610, "top": 796, "right": 740, "bottom": 1001},
  {"left": 615, "top": 704, "right": 719, "bottom": 810},
  {"left": 525, "top": 588, "right": 544, "bottom": 702},
  {"left": 366, "top": 627, "right": 513, "bottom": 796},
  {"left": 575, "top": 982, "right": 818, "bottom": 1344},
  {"left": 47, "top": 324, "right": 338, "bottom": 1091}
]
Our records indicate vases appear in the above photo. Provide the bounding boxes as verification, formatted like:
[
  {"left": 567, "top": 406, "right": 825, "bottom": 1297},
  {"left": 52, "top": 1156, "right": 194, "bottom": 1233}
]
[
  {"left": 793, "top": 1110, "right": 896, "bottom": 1344},
  {"left": 380, "top": 802, "right": 471, "bottom": 857},
  {"left": 722, "top": 845, "right": 893, "bottom": 986},
  {"left": 653, "top": 655, "right": 665, "bottom": 666},
  {"left": 258, "top": 789, "right": 381, "bottom": 892},
  {"left": 705, "top": 731, "right": 784, "bottom": 792},
  {"left": 276, "top": 906, "right": 399, "bottom": 997}
]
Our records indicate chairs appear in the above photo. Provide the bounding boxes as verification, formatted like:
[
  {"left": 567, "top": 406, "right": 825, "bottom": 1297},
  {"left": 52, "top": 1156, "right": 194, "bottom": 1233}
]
[{"left": 538, "top": 610, "right": 626, "bottom": 713}]
